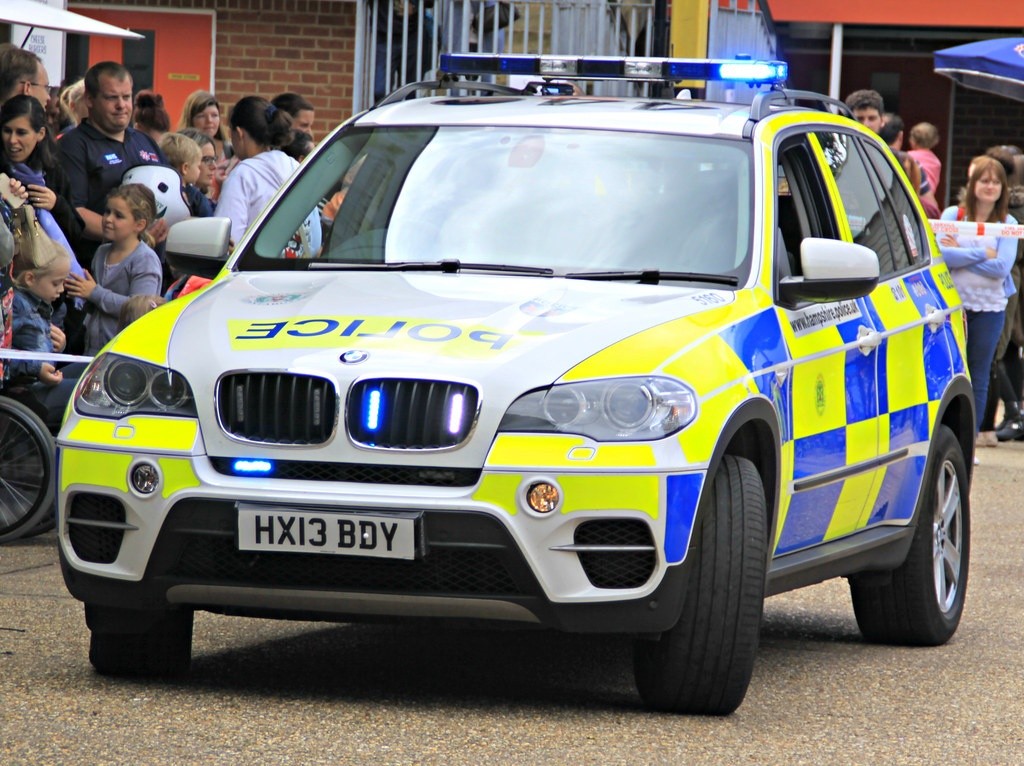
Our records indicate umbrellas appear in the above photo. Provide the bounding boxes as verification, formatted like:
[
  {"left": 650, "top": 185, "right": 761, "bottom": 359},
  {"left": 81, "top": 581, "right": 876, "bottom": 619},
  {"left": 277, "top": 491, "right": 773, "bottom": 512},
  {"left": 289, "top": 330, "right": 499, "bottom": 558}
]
[{"left": 934, "top": 37, "right": 1024, "bottom": 104}]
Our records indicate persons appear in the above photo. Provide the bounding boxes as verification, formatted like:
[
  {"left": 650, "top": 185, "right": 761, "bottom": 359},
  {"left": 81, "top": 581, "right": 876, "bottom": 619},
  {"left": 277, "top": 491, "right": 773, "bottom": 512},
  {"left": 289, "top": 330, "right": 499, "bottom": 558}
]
[
  {"left": 281, "top": 127, "right": 373, "bottom": 228},
  {"left": 64, "top": 182, "right": 163, "bottom": 359},
  {"left": 0, "top": 42, "right": 87, "bottom": 368},
  {"left": 368, "top": 0, "right": 505, "bottom": 109},
  {"left": 134, "top": 90, "right": 242, "bottom": 219},
  {"left": 0, "top": 237, "right": 95, "bottom": 439},
  {"left": 117, "top": 294, "right": 166, "bottom": 334},
  {"left": 934, "top": 141, "right": 1024, "bottom": 468},
  {"left": 846, "top": 89, "right": 942, "bottom": 217},
  {"left": 46, "top": 62, "right": 192, "bottom": 294},
  {"left": 213, "top": 93, "right": 323, "bottom": 261},
  {"left": 272, "top": 92, "right": 315, "bottom": 135}
]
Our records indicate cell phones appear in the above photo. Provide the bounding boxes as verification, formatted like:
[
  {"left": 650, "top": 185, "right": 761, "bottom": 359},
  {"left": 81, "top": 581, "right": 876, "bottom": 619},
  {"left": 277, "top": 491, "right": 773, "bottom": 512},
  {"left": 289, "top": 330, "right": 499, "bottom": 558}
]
[{"left": 0, "top": 173, "right": 26, "bottom": 209}]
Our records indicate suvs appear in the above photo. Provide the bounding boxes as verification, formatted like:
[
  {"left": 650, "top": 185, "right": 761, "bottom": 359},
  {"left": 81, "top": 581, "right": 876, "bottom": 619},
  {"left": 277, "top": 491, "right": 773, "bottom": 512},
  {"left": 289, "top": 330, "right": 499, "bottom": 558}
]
[{"left": 53, "top": 50, "right": 978, "bottom": 718}]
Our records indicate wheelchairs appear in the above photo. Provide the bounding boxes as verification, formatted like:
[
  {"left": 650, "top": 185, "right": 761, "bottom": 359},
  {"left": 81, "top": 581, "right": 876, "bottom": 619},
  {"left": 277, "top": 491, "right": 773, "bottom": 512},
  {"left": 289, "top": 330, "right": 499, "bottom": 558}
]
[{"left": 0, "top": 370, "right": 57, "bottom": 543}]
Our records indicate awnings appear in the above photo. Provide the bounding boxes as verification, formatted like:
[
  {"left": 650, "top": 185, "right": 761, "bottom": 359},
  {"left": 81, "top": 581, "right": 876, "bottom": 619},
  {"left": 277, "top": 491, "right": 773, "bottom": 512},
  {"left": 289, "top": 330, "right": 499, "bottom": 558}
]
[{"left": 0, "top": 0, "right": 147, "bottom": 52}]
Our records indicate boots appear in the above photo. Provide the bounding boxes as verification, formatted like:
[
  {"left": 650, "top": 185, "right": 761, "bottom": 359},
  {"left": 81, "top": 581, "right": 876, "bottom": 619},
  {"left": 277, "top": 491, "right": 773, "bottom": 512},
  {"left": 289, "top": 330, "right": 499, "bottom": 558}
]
[{"left": 994, "top": 401, "right": 1024, "bottom": 442}]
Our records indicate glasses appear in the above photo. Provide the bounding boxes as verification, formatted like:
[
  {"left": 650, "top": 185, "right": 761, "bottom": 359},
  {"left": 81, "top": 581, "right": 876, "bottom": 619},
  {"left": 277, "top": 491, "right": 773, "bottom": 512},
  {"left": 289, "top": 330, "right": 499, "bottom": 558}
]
[
  {"left": 18, "top": 81, "right": 52, "bottom": 93},
  {"left": 201, "top": 155, "right": 217, "bottom": 165}
]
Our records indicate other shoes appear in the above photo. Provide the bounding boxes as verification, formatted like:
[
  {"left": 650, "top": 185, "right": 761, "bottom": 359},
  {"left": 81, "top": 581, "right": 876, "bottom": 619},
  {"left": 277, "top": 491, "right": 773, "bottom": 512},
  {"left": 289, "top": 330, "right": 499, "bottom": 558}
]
[{"left": 974, "top": 432, "right": 997, "bottom": 447}]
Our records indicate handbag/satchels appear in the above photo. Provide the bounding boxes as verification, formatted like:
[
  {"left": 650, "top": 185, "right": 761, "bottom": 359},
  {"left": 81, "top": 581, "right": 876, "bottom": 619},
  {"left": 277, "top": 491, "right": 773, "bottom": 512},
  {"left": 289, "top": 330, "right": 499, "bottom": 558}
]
[{"left": 9, "top": 205, "right": 57, "bottom": 269}]
